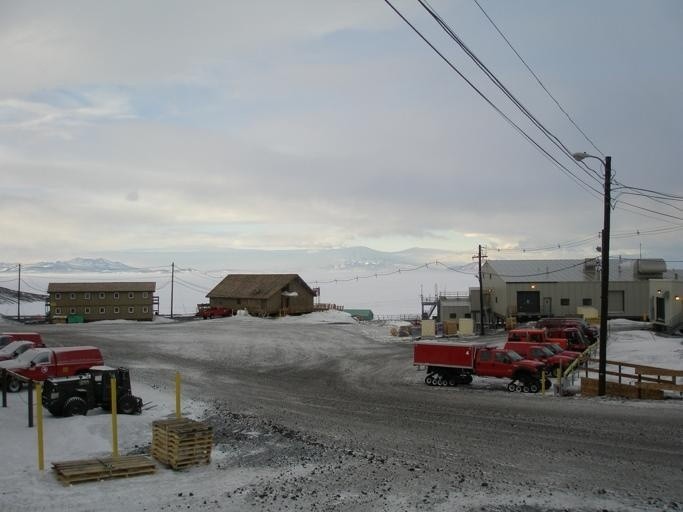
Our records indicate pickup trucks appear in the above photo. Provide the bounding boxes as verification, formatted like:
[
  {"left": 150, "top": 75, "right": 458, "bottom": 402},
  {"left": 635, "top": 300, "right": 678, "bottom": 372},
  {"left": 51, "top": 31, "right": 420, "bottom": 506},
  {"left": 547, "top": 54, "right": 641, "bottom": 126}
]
[{"left": 200, "top": 304, "right": 230, "bottom": 320}]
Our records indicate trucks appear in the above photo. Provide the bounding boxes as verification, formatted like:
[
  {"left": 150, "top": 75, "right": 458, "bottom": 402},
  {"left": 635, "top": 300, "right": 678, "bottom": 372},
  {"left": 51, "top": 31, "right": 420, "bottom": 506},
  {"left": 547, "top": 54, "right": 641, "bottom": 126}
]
[
  {"left": 0, "top": 330, "right": 104, "bottom": 394},
  {"left": 411, "top": 340, "right": 552, "bottom": 395}
]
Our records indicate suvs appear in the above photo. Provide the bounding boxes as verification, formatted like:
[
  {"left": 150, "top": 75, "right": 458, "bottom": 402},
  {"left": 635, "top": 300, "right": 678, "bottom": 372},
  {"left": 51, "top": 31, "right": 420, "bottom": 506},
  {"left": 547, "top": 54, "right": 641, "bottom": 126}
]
[{"left": 505, "top": 315, "right": 598, "bottom": 379}]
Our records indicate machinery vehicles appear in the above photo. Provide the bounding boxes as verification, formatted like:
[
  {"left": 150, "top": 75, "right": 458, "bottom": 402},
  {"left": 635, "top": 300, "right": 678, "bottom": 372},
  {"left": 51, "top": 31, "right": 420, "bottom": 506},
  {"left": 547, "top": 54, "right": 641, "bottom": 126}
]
[{"left": 40, "top": 360, "right": 144, "bottom": 418}]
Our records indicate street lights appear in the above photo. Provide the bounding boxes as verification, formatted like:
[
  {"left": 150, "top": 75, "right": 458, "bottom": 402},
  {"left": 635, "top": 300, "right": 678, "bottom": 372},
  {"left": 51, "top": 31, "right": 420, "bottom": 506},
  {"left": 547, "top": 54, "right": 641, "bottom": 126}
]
[
  {"left": 568, "top": 148, "right": 612, "bottom": 396},
  {"left": 473, "top": 274, "right": 484, "bottom": 335}
]
